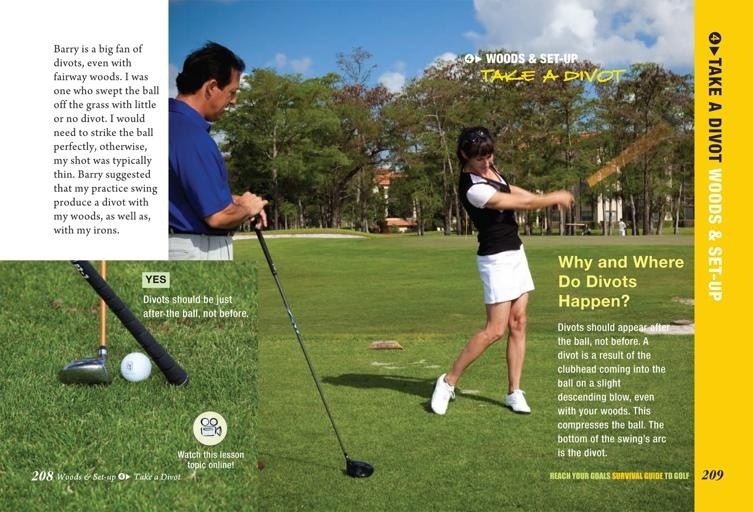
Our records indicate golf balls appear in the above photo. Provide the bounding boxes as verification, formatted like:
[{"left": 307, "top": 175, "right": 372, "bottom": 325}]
[{"left": 120, "top": 351, "right": 152, "bottom": 381}]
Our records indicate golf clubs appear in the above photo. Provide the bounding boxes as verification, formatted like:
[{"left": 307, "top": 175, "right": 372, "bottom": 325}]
[
  {"left": 60, "top": 259, "right": 112, "bottom": 385},
  {"left": 250, "top": 215, "right": 375, "bottom": 478}
]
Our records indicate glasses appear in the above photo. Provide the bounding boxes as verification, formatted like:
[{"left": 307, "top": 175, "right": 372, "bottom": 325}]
[{"left": 462, "top": 127, "right": 489, "bottom": 141}]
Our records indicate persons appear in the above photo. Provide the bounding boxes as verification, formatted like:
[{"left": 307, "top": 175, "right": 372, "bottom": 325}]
[
  {"left": 619, "top": 219, "right": 627, "bottom": 236},
  {"left": 169, "top": 41, "right": 269, "bottom": 261},
  {"left": 431, "top": 127, "right": 576, "bottom": 415}
]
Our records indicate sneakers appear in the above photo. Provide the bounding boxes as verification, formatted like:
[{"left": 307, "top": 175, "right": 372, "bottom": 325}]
[
  {"left": 431, "top": 374, "right": 455, "bottom": 415},
  {"left": 506, "top": 390, "right": 531, "bottom": 413}
]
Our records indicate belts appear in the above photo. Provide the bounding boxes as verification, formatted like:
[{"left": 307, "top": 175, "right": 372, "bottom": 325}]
[{"left": 169, "top": 227, "right": 234, "bottom": 236}]
